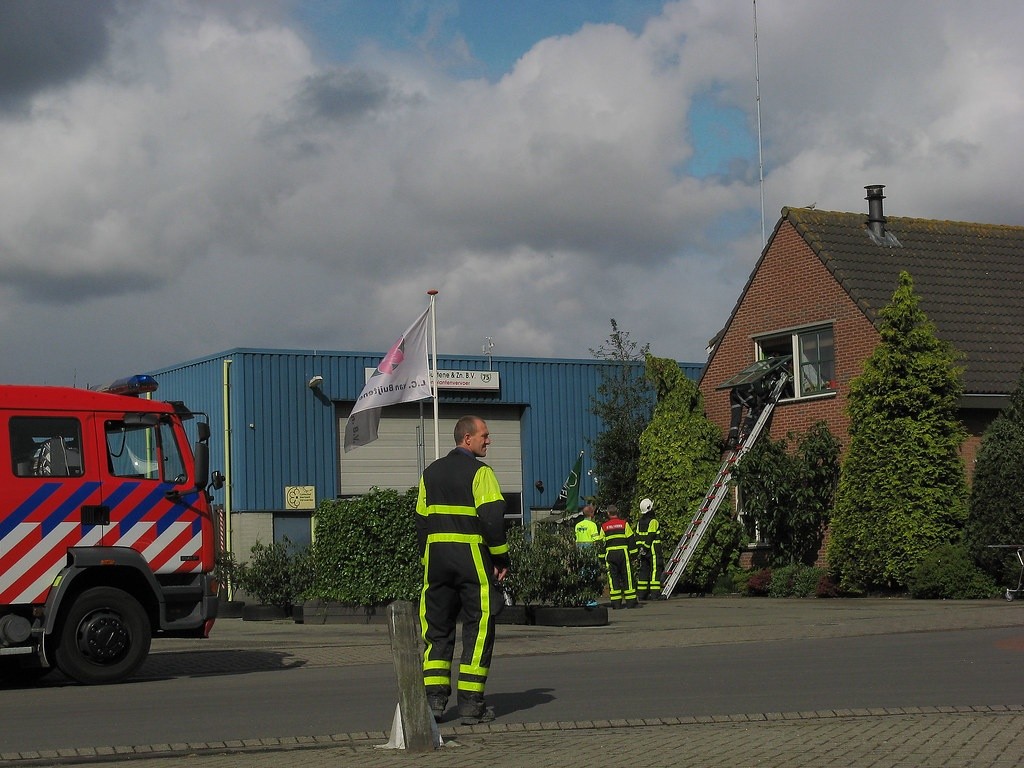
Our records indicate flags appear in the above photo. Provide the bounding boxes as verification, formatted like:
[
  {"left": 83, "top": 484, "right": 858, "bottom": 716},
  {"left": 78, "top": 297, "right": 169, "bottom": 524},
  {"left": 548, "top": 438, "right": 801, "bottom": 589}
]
[
  {"left": 342, "top": 308, "right": 432, "bottom": 454},
  {"left": 549, "top": 454, "right": 583, "bottom": 514}
]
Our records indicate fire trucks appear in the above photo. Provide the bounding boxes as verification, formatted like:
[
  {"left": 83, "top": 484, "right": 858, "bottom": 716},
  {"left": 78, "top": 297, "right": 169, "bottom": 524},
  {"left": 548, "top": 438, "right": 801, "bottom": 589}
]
[{"left": 1, "top": 375, "right": 224, "bottom": 687}]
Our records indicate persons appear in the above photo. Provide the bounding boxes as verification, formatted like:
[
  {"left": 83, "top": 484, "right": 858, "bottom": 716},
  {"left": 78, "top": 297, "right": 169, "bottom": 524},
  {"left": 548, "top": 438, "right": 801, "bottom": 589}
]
[
  {"left": 726, "top": 367, "right": 780, "bottom": 448},
  {"left": 636, "top": 498, "right": 667, "bottom": 601},
  {"left": 575, "top": 505, "right": 601, "bottom": 550},
  {"left": 412, "top": 413, "right": 510, "bottom": 722},
  {"left": 599, "top": 505, "right": 638, "bottom": 609}
]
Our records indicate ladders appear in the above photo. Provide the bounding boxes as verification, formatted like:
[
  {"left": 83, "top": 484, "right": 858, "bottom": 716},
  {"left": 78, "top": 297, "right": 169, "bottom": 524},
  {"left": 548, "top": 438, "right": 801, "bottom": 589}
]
[{"left": 660, "top": 371, "right": 789, "bottom": 600}]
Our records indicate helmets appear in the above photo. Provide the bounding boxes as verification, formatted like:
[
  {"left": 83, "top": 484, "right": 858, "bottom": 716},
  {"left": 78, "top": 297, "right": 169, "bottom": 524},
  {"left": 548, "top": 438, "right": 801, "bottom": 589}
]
[{"left": 639, "top": 498, "right": 654, "bottom": 514}]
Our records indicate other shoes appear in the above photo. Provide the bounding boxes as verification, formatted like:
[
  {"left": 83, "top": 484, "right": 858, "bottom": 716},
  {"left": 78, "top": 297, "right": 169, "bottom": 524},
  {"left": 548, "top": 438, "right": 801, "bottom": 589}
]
[
  {"left": 639, "top": 595, "right": 651, "bottom": 600},
  {"left": 460, "top": 709, "right": 495, "bottom": 724},
  {"left": 627, "top": 602, "right": 642, "bottom": 608},
  {"left": 652, "top": 594, "right": 667, "bottom": 600},
  {"left": 613, "top": 603, "right": 626, "bottom": 609},
  {"left": 433, "top": 709, "right": 444, "bottom": 722}
]
